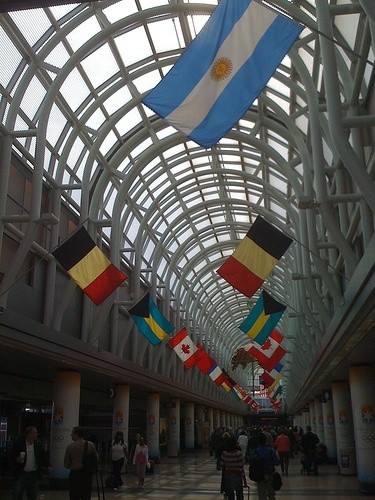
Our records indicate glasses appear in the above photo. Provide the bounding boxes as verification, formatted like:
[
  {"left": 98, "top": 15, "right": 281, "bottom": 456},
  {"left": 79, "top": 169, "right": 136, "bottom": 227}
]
[{"left": 224, "top": 436, "right": 229, "bottom": 438}]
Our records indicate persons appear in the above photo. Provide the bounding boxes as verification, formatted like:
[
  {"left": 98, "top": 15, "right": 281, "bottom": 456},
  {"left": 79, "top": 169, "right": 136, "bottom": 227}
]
[
  {"left": 11, "top": 426, "right": 53, "bottom": 500},
  {"left": 111, "top": 431, "right": 130, "bottom": 491},
  {"left": 275, "top": 430, "right": 291, "bottom": 476},
  {"left": 209, "top": 423, "right": 303, "bottom": 471},
  {"left": 219, "top": 437, "right": 247, "bottom": 500},
  {"left": 249, "top": 433, "right": 281, "bottom": 500},
  {"left": 130, "top": 433, "right": 152, "bottom": 488},
  {"left": 301, "top": 426, "right": 321, "bottom": 476},
  {"left": 64, "top": 426, "right": 100, "bottom": 500}
]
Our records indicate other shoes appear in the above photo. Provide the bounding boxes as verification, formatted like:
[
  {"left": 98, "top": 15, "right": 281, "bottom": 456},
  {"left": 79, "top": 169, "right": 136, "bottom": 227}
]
[
  {"left": 314, "top": 472, "right": 319, "bottom": 476},
  {"left": 114, "top": 488, "right": 118, "bottom": 491},
  {"left": 307, "top": 472, "right": 310, "bottom": 476},
  {"left": 138, "top": 484, "right": 144, "bottom": 488}
]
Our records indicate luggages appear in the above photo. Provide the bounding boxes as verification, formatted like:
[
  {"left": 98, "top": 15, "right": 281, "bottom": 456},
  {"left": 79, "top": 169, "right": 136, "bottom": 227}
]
[
  {"left": 105, "top": 462, "right": 115, "bottom": 489},
  {"left": 146, "top": 460, "right": 154, "bottom": 474},
  {"left": 274, "top": 470, "right": 281, "bottom": 490}
]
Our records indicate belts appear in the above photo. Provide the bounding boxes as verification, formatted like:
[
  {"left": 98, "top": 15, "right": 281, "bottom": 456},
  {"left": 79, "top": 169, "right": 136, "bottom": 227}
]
[{"left": 23, "top": 471, "right": 37, "bottom": 474}]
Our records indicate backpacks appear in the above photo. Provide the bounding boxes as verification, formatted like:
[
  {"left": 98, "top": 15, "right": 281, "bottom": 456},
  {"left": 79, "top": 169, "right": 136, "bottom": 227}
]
[{"left": 249, "top": 449, "right": 261, "bottom": 482}]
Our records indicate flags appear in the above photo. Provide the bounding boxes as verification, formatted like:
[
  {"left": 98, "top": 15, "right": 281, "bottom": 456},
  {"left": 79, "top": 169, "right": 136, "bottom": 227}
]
[
  {"left": 195, "top": 343, "right": 260, "bottom": 412},
  {"left": 217, "top": 215, "right": 293, "bottom": 299},
  {"left": 127, "top": 291, "right": 175, "bottom": 347},
  {"left": 52, "top": 226, "right": 127, "bottom": 306},
  {"left": 238, "top": 289, "right": 287, "bottom": 346},
  {"left": 243, "top": 330, "right": 286, "bottom": 414},
  {"left": 140, "top": 1, "right": 305, "bottom": 148},
  {"left": 168, "top": 327, "right": 203, "bottom": 369}
]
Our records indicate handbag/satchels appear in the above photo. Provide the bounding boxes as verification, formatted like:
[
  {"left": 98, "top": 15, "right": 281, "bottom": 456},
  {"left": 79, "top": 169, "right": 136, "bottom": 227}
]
[{"left": 83, "top": 441, "right": 98, "bottom": 475}]
